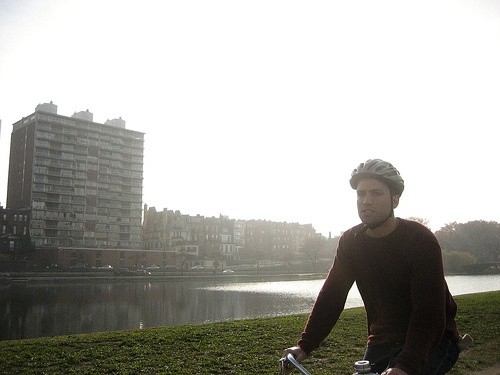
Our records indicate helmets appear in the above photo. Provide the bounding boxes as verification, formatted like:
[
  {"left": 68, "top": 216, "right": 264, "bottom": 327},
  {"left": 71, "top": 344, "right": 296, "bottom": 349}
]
[{"left": 350, "top": 159, "right": 404, "bottom": 197}]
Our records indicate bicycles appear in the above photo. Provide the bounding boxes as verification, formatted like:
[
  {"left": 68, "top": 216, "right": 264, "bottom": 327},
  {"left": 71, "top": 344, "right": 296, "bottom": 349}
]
[{"left": 282, "top": 352, "right": 382, "bottom": 375}]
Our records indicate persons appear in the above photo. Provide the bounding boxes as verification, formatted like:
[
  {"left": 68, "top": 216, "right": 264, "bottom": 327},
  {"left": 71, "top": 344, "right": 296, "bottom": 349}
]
[{"left": 281, "top": 159, "right": 461, "bottom": 375}]
